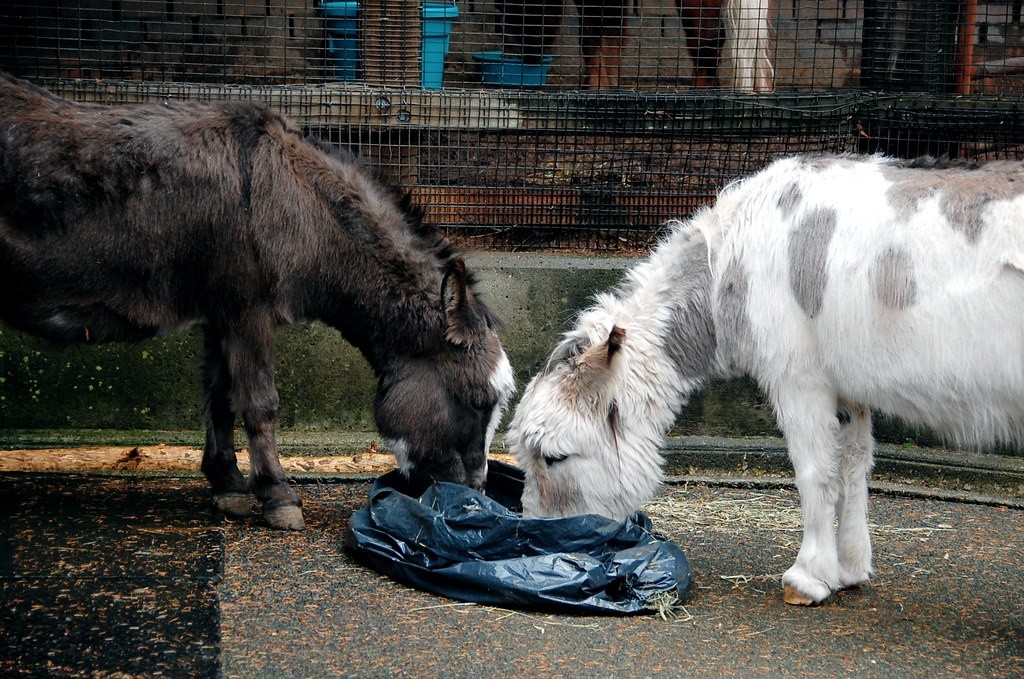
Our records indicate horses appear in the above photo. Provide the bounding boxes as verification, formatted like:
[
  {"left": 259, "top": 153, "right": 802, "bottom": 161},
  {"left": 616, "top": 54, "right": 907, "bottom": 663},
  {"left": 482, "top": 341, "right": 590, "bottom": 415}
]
[
  {"left": 0, "top": 75, "right": 515, "bottom": 532},
  {"left": 493, "top": 0, "right": 778, "bottom": 92},
  {"left": 504, "top": 153, "right": 1024, "bottom": 606}
]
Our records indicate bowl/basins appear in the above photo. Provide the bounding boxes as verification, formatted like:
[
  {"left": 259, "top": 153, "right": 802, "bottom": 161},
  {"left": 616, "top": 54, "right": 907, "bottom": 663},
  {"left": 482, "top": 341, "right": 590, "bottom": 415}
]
[{"left": 472, "top": 50, "right": 553, "bottom": 87}]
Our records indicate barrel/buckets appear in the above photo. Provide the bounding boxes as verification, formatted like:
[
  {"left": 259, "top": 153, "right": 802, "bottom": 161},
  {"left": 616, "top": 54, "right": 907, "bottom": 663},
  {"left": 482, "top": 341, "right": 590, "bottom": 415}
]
[{"left": 321, "top": 1, "right": 457, "bottom": 89}]
